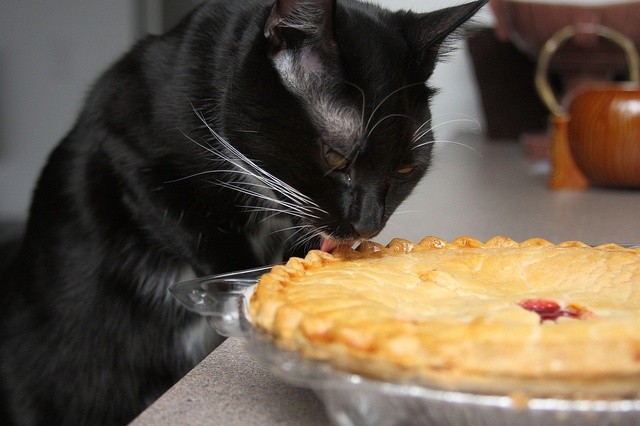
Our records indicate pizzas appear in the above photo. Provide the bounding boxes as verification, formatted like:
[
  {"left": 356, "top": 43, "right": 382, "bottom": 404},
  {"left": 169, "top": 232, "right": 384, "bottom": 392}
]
[{"left": 259, "top": 236, "right": 640, "bottom": 388}]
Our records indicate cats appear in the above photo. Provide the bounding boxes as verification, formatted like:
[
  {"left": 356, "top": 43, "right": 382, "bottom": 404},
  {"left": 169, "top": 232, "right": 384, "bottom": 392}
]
[{"left": 0, "top": 0, "right": 487, "bottom": 426}]
